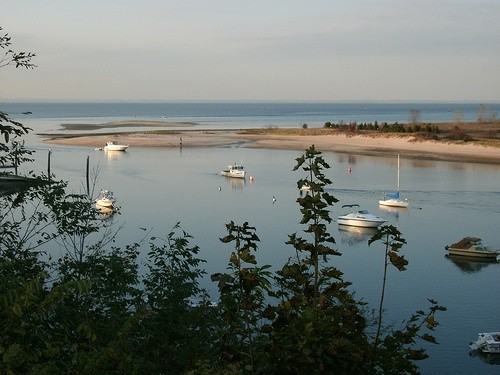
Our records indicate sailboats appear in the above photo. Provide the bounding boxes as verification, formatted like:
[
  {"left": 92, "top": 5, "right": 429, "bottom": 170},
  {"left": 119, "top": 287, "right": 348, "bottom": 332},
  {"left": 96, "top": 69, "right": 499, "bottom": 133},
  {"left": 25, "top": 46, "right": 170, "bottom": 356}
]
[{"left": 378, "top": 153, "right": 409, "bottom": 207}]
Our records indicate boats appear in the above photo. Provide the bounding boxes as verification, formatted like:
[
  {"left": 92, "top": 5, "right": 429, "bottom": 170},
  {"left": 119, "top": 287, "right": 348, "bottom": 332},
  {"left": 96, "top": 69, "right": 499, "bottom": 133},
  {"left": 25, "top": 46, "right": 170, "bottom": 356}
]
[
  {"left": 104, "top": 141, "right": 129, "bottom": 151},
  {"left": 221, "top": 163, "right": 245, "bottom": 179},
  {"left": 469, "top": 332, "right": 500, "bottom": 353},
  {"left": 95, "top": 189, "right": 115, "bottom": 206},
  {"left": 337, "top": 204, "right": 388, "bottom": 228},
  {"left": 445, "top": 237, "right": 500, "bottom": 258}
]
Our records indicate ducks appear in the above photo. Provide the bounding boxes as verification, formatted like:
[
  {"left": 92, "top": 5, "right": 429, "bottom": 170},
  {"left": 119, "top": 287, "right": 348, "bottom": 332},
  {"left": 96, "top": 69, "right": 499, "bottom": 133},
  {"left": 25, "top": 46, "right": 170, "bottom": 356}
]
[
  {"left": 271, "top": 195, "right": 277, "bottom": 204},
  {"left": 216, "top": 185, "right": 223, "bottom": 192}
]
